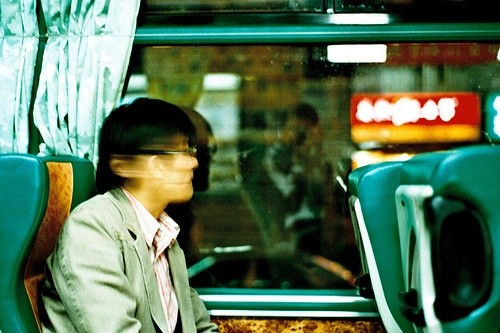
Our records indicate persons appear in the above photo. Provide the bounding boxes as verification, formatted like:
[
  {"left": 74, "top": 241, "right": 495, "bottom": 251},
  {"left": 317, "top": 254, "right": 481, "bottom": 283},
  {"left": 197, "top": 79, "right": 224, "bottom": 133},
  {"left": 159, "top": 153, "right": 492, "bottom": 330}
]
[
  {"left": 166, "top": 103, "right": 355, "bottom": 296},
  {"left": 37, "top": 98, "right": 221, "bottom": 333}
]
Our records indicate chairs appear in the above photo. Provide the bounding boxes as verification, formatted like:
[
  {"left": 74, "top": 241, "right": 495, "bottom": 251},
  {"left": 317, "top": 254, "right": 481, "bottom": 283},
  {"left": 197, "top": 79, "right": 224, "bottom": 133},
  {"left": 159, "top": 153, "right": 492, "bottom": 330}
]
[
  {"left": 233, "top": 127, "right": 296, "bottom": 258},
  {"left": 342, "top": 143, "right": 500, "bottom": 333},
  {"left": 0, "top": 153, "right": 94, "bottom": 333}
]
